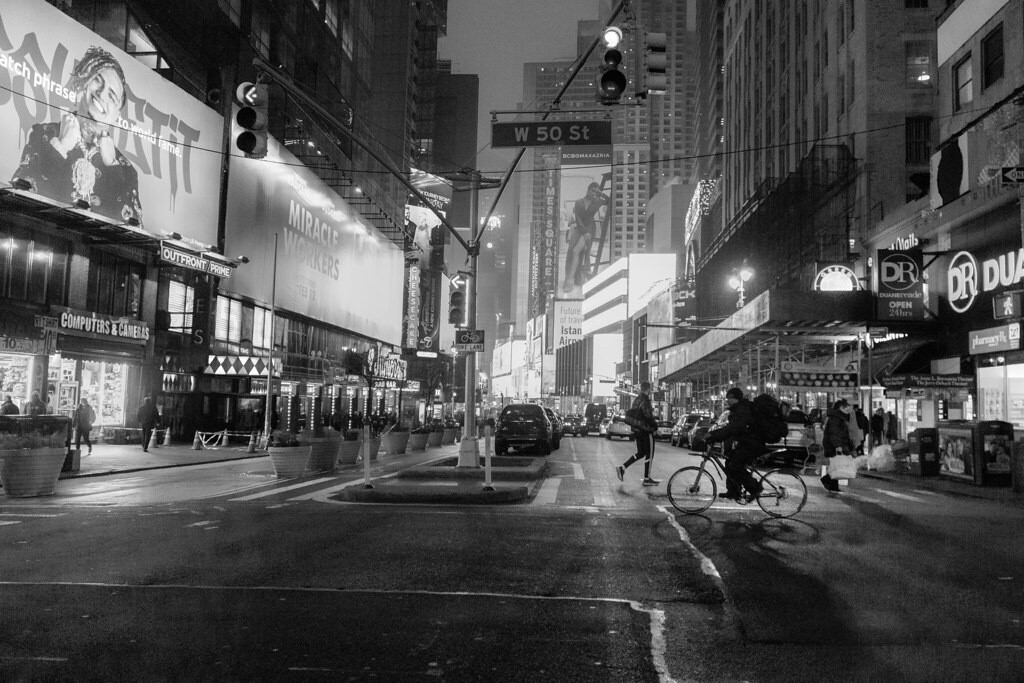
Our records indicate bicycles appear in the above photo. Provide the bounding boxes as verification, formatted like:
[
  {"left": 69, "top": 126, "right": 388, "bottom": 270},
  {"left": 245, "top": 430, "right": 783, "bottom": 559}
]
[{"left": 666, "top": 445, "right": 807, "bottom": 518}]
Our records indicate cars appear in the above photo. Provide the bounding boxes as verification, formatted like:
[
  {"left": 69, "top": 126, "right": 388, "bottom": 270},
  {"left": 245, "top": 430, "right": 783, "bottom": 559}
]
[
  {"left": 653, "top": 420, "right": 676, "bottom": 442},
  {"left": 563, "top": 414, "right": 585, "bottom": 438},
  {"left": 599, "top": 418, "right": 611, "bottom": 436},
  {"left": 687, "top": 418, "right": 716, "bottom": 450},
  {"left": 545, "top": 408, "right": 563, "bottom": 450}
]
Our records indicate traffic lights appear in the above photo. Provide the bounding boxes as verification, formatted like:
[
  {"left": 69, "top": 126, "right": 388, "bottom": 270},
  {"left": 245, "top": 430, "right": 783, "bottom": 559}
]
[
  {"left": 602, "top": 26, "right": 627, "bottom": 105},
  {"left": 447, "top": 275, "right": 466, "bottom": 325},
  {"left": 644, "top": 32, "right": 668, "bottom": 91},
  {"left": 234, "top": 81, "right": 268, "bottom": 158}
]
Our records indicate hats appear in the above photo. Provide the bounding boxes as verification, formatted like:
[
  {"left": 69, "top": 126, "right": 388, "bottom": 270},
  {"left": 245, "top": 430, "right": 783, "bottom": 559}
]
[{"left": 726, "top": 388, "right": 743, "bottom": 399}]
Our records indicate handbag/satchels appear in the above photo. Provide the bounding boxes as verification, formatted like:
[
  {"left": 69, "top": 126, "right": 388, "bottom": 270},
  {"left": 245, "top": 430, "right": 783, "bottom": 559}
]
[
  {"left": 624, "top": 396, "right": 658, "bottom": 434},
  {"left": 829, "top": 450, "right": 858, "bottom": 478},
  {"left": 788, "top": 410, "right": 808, "bottom": 423}
]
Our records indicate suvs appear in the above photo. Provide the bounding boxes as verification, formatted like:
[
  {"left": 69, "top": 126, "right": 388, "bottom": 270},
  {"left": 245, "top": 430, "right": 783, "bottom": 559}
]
[
  {"left": 606, "top": 409, "right": 635, "bottom": 441},
  {"left": 706, "top": 410, "right": 738, "bottom": 448},
  {"left": 671, "top": 413, "right": 712, "bottom": 447},
  {"left": 495, "top": 402, "right": 554, "bottom": 456},
  {"left": 763, "top": 407, "right": 816, "bottom": 464}
]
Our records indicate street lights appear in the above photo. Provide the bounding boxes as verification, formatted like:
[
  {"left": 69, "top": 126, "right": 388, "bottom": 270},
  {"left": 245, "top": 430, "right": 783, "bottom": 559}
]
[{"left": 729, "top": 269, "right": 753, "bottom": 309}]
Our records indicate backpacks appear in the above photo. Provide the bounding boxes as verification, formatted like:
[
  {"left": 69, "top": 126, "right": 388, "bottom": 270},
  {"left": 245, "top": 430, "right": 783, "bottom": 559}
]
[{"left": 750, "top": 393, "right": 788, "bottom": 443}]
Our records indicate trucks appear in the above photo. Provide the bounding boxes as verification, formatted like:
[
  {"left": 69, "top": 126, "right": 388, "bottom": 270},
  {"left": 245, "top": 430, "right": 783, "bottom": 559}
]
[{"left": 586, "top": 403, "right": 607, "bottom": 434}]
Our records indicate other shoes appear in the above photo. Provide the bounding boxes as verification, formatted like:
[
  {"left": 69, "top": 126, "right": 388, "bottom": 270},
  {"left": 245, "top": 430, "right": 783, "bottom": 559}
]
[
  {"left": 719, "top": 490, "right": 742, "bottom": 498},
  {"left": 747, "top": 480, "right": 764, "bottom": 502},
  {"left": 829, "top": 486, "right": 841, "bottom": 491},
  {"left": 821, "top": 477, "right": 830, "bottom": 490}
]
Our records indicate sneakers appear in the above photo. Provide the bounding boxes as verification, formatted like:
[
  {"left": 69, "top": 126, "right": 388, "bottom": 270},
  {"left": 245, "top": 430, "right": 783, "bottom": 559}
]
[
  {"left": 642, "top": 478, "right": 660, "bottom": 485},
  {"left": 616, "top": 466, "right": 624, "bottom": 482}
]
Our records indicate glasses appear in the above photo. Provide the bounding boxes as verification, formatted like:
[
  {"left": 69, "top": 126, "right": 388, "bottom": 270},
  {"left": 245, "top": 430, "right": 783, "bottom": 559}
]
[{"left": 726, "top": 396, "right": 737, "bottom": 399}]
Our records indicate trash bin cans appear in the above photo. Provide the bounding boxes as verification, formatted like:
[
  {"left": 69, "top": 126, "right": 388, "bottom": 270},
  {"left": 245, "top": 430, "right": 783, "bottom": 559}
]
[
  {"left": 935, "top": 418, "right": 1015, "bottom": 487},
  {"left": 906, "top": 427, "right": 938, "bottom": 477}
]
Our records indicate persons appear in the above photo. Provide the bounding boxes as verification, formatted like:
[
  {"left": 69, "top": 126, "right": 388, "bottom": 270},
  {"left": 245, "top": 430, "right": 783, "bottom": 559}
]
[
  {"left": 24, "top": 392, "right": 46, "bottom": 415},
  {"left": 14, "top": 47, "right": 145, "bottom": 229},
  {"left": 0, "top": 395, "right": 19, "bottom": 414},
  {"left": 137, "top": 398, "right": 160, "bottom": 452},
  {"left": 617, "top": 381, "right": 660, "bottom": 485},
  {"left": 563, "top": 182, "right": 609, "bottom": 292},
  {"left": 789, "top": 399, "right": 896, "bottom": 492},
  {"left": 240, "top": 404, "right": 256, "bottom": 445},
  {"left": 73, "top": 398, "right": 96, "bottom": 455},
  {"left": 704, "top": 387, "right": 765, "bottom": 503},
  {"left": 324, "top": 410, "right": 396, "bottom": 436}
]
